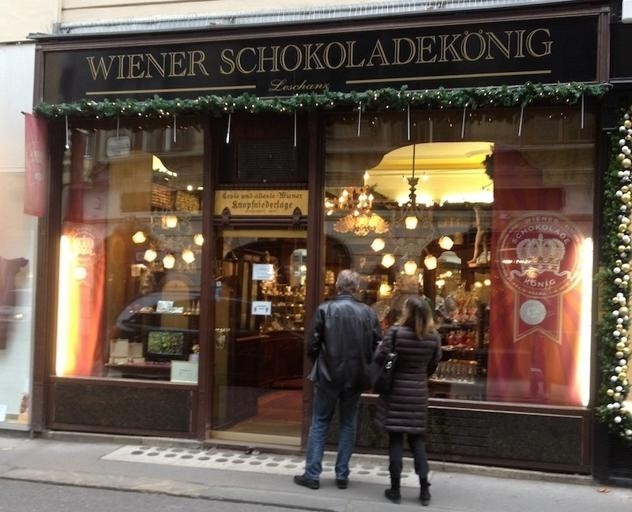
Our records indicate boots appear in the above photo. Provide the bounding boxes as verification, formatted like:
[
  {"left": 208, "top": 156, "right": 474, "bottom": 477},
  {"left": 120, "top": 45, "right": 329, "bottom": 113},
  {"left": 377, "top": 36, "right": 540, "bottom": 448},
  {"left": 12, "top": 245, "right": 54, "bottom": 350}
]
[
  {"left": 419, "top": 477, "right": 430, "bottom": 505},
  {"left": 385, "top": 473, "right": 400, "bottom": 503}
]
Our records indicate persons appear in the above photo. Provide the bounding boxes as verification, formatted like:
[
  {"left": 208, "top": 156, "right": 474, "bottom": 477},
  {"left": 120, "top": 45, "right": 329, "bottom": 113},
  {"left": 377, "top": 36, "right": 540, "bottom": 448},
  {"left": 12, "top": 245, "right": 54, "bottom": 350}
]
[
  {"left": 294, "top": 268, "right": 383, "bottom": 489},
  {"left": 374, "top": 296, "right": 442, "bottom": 506}
]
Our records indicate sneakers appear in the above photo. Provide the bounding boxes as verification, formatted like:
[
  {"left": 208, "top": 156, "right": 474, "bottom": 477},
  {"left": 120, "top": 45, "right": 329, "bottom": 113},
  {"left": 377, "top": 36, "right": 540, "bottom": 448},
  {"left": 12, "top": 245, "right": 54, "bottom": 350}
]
[
  {"left": 295, "top": 474, "right": 319, "bottom": 489},
  {"left": 338, "top": 479, "right": 348, "bottom": 488}
]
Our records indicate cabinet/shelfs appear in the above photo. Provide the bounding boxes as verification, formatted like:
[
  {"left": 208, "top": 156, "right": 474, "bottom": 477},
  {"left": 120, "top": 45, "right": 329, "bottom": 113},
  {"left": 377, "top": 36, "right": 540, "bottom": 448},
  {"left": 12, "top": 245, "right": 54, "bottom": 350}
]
[{"left": 213, "top": 250, "right": 270, "bottom": 431}]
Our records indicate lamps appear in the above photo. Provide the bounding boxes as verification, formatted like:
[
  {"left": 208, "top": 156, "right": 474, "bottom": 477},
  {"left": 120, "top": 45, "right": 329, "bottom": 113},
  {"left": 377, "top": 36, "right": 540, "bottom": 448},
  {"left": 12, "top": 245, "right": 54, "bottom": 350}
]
[
  {"left": 364, "top": 109, "right": 455, "bottom": 276},
  {"left": 129, "top": 124, "right": 205, "bottom": 269}
]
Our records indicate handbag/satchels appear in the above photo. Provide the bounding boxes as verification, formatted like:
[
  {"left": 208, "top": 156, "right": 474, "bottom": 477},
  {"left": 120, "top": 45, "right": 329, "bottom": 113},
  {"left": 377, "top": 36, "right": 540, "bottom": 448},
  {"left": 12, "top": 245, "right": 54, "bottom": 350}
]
[{"left": 371, "top": 341, "right": 398, "bottom": 393}]
